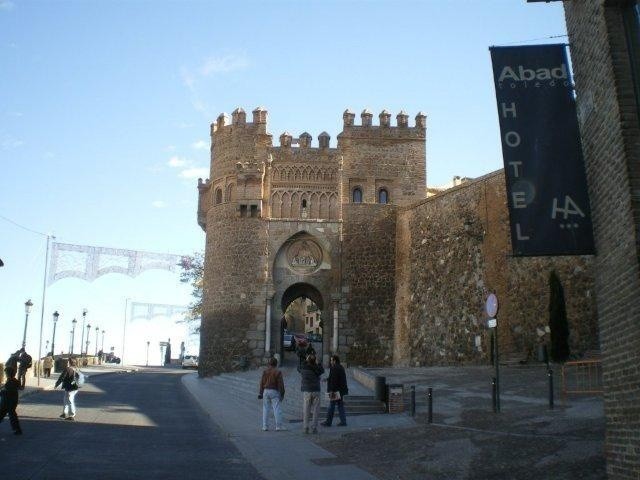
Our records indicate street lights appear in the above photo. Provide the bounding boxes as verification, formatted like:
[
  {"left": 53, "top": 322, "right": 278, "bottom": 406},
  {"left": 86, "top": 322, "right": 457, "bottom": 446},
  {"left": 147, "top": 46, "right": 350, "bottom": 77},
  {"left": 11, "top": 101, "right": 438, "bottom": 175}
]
[
  {"left": 69, "top": 308, "right": 172, "bottom": 366},
  {"left": 51, "top": 311, "right": 59, "bottom": 355},
  {"left": 20, "top": 299, "right": 34, "bottom": 350}
]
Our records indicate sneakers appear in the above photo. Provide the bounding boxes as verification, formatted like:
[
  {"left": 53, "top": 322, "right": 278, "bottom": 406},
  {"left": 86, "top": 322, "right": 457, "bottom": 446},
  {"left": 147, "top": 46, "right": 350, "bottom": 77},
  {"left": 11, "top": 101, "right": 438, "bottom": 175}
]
[{"left": 262, "top": 421, "right": 347, "bottom": 434}]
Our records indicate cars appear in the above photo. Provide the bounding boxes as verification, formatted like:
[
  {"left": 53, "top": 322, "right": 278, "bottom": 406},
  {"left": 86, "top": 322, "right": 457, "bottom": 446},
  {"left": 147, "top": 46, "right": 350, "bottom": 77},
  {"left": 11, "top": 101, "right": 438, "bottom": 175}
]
[
  {"left": 181, "top": 355, "right": 198, "bottom": 370},
  {"left": 281, "top": 331, "right": 322, "bottom": 352}
]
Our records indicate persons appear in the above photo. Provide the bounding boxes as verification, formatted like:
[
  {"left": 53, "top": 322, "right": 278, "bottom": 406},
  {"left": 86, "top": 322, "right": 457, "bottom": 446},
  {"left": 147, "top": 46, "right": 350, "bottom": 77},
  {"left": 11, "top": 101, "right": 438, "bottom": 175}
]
[
  {"left": 258, "top": 358, "right": 288, "bottom": 431},
  {"left": 5, "top": 350, "right": 21, "bottom": 378},
  {"left": 98, "top": 349, "right": 103, "bottom": 366},
  {"left": 0, "top": 367, "right": 23, "bottom": 435},
  {"left": 297, "top": 355, "right": 324, "bottom": 434},
  {"left": 320, "top": 355, "right": 348, "bottom": 426},
  {"left": 289, "top": 336, "right": 317, "bottom": 365},
  {"left": 18, "top": 348, "right": 32, "bottom": 390},
  {"left": 54, "top": 358, "right": 80, "bottom": 421},
  {"left": 43, "top": 352, "right": 53, "bottom": 378}
]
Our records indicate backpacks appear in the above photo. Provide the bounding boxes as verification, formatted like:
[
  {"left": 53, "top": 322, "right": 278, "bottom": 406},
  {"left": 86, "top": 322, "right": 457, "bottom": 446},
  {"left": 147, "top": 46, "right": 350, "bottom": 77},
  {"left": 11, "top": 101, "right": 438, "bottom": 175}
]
[{"left": 67, "top": 366, "right": 85, "bottom": 390}]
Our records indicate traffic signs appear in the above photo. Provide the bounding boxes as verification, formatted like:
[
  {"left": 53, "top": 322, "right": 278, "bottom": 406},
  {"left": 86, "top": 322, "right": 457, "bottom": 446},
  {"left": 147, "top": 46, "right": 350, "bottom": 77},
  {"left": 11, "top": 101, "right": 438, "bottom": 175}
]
[{"left": 488, "top": 320, "right": 499, "bottom": 328}]
